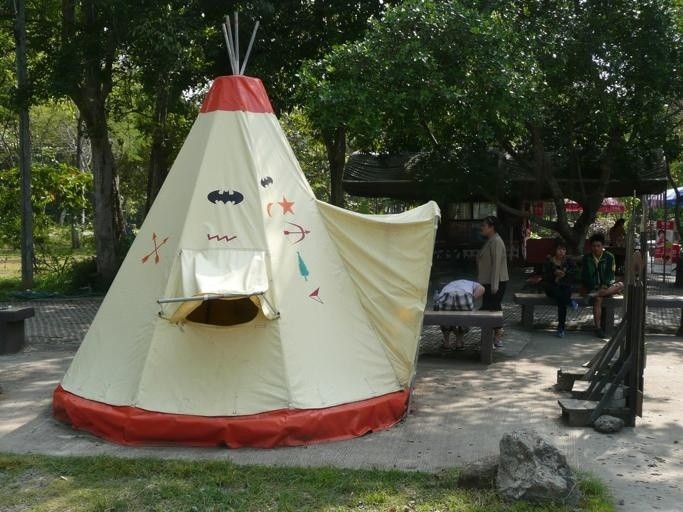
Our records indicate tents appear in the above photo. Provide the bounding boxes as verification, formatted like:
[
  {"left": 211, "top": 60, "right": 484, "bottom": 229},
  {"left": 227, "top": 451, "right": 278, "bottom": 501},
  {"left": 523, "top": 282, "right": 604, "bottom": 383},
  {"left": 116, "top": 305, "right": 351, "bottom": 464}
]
[{"left": 45, "top": 71, "right": 442, "bottom": 448}]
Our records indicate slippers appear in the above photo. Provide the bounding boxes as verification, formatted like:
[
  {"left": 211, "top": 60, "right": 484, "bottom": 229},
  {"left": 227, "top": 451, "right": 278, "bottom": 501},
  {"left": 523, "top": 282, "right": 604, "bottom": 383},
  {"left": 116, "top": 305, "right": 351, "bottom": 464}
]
[{"left": 439, "top": 340, "right": 503, "bottom": 351}]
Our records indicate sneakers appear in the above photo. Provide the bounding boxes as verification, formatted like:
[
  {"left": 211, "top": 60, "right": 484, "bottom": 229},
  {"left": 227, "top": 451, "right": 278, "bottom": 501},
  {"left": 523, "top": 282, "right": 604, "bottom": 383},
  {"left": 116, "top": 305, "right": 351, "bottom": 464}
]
[
  {"left": 570, "top": 301, "right": 579, "bottom": 310},
  {"left": 558, "top": 326, "right": 565, "bottom": 337},
  {"left": 596, "top": 328, "right": 604, "bottom": 338},
  {"left": 583, "top": 294, "right": 592, "bottom": 306}
]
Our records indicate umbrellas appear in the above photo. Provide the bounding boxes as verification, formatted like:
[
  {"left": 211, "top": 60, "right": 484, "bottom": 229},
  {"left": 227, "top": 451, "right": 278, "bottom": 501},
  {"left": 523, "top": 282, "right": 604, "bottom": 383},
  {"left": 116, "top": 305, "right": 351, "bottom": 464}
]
[{"left": 565, "top": 198, "right": 624, "bottom": 213}]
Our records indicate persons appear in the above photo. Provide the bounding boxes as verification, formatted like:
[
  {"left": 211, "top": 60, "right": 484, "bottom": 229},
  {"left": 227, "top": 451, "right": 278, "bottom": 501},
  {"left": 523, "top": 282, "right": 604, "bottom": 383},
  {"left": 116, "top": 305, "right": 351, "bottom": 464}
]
[
  {"left": 474, "top": 216, "right": 509, "bottom": 345},
  {"left": 611, "top": 217, "right": 627, "bottom": 274},
  {"left": 432, "top": 279, "right": 485, "bottom": 348},
  {"left": 658, "top": 230, "right": 670, "bottom": 244},
  {"left": 582, "top": 235, "right": 625, "bottom": 337},
  {"left": 541, "top": 242, "right": 580, "bottom": 337}
]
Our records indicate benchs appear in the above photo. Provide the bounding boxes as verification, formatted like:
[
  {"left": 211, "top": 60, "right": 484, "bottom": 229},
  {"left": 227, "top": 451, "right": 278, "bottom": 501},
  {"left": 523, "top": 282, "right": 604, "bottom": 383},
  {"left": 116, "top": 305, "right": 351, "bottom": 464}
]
[
  {"left": 514, "top": 294, "right": 683, "bottom": 335},
  {"left": 423, "top": 309, "right": 503, "bottom": 363}
]
